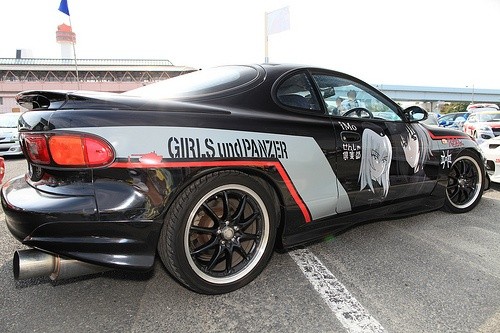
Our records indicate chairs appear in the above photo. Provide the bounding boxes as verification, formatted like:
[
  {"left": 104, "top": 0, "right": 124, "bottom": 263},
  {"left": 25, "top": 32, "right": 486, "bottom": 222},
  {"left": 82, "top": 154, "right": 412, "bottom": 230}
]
[{"left": 279, "top": 94, "right": 310, "bottom": 111}]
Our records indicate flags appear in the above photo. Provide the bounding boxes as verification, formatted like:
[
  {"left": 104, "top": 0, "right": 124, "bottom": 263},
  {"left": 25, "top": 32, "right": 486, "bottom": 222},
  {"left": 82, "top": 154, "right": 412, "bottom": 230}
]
[{"left": 58, "top": 0, "right": 70, "bottom": 16}]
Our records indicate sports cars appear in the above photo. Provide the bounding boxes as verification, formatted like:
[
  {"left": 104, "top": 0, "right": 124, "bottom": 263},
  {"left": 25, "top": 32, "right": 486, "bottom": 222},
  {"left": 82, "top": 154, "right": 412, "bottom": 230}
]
[{"left": 0, "top": 62, "right": 491, "bottom": 294}]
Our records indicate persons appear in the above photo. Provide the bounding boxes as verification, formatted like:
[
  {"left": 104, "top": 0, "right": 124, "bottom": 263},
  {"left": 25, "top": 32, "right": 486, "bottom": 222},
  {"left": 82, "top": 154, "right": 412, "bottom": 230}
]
[{"left": 332, "top": 87, "right": 368, "bottom": 117}]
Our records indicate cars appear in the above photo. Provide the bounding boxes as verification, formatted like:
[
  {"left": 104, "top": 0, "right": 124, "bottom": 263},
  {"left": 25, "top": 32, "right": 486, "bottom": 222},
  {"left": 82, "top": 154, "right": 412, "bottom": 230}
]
[
  {"left": 466, "top": 103, "right": 499, "bottom": 113},
  {"left": 478, "top": 135, "right": 500, "bottom": 183},
  {"left": 0, "top": 112, "right": 25, "bottom": 156},
  {"left": 463, "top": 111, "right": 500, "bottom": 145},
  {"left": 436, "top": 112, "right": 471, "bottom": 128}
]
